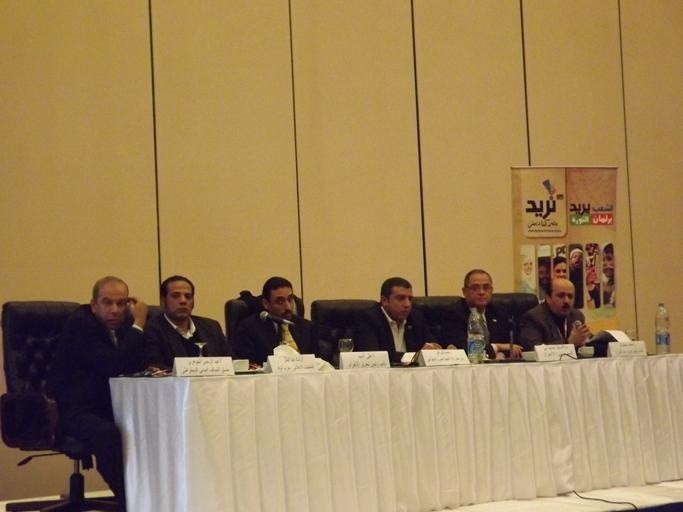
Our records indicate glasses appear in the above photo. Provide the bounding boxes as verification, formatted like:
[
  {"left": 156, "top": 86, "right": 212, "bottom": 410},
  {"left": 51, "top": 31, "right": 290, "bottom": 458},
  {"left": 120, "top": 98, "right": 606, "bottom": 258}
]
[{"left": 466, "top": 284, "right": 494, "bottom": 293}]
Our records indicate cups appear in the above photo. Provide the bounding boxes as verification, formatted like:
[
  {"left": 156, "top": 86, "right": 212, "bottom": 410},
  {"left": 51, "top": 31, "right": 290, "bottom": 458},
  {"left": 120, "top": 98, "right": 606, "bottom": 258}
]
[
  {"left": 338, "top": 338, "right": 354, "bottom": 352},
  {"left": 232, "top": 359, "right": 251, "bottom": 369},
  {"left": 625, "top": 330, "right": 636, "bottom": 340},
  {"left": 195, "top": 341, "right": 208, "bottom": 357},
  {"left": 521, "top": 352, "right": 538, "bottom": 363},
  {"left": 577, "top": 346, "right": 596, "bottom": 358}
]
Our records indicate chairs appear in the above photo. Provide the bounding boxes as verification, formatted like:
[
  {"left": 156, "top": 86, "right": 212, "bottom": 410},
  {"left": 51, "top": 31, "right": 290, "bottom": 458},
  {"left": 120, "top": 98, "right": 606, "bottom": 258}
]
[
  {"left": 310, "top": 299, "right": 380, "bottom": 349},
  {"left": 224, "top": 296, "right": 305, "bottom": 350},
  {"left": 411, "top": 296, "right": 466, "bottom": 344},
  {"left": 492, "top": 293, "right": 540, "bottom": 336},
  {"left": 146, "top": 305, "right": 167, "bottom": 327},
  {"left": 0, "top": 301, "right": 118, "bottom": 512}
]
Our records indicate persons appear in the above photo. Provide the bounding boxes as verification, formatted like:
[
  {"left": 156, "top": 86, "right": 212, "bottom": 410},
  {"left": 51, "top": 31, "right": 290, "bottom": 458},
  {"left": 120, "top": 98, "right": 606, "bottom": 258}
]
[
  {"left": 45, "top": 276, "right": 155, "bottom": 511},
  {"left": 554, "top": 255, "right": 567, "bottom": 282},
  {"left": 538, "top": 258, "right": 551, "bottom": 300},
  {"left": 348, "top": 278, "right": 456, "bottom": 368},
  {"left": 520, "top": 253, "right": 536, "bottom": 299},
  {"left": 569, "top": 249, "right": 583, "bottom": 309},
  {"left": 586, "top": 243, "right": 600, "bottom": 307},
  {"left": 439, "top": 270, "right": 522, "bottom": 367},
  {"left": 232, "top": 277, "right": 320, "bottom": 370},
  {"left": 602, "top": 243, "right": 615, "bottom": 306},
  {"left": 145, "top": 276, "right": 227, "bottom": 375},
  {"left": 520, "top": 281, "right": 594, "bottom": 359}
]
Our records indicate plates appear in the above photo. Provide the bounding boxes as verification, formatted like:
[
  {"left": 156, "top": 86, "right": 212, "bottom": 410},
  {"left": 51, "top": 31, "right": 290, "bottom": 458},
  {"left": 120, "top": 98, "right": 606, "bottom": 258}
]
[{"left": 235, "top": 366, "right": 263, "bottom": 374}]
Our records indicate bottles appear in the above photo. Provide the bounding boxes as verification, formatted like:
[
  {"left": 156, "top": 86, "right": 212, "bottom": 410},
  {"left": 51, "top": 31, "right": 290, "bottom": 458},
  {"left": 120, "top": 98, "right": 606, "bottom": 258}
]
[
  {"left": 467, "top": 308, "right": 486, "bottom": 364},
  {"left": 655, "top": 304, "right": 670, "bottom": 356}
]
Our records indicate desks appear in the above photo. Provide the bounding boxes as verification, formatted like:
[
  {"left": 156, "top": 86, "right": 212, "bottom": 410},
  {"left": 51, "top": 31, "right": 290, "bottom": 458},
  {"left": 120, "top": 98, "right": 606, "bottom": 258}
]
[{"left": 108, "top": 351, "right": 683, "bottom": 512}]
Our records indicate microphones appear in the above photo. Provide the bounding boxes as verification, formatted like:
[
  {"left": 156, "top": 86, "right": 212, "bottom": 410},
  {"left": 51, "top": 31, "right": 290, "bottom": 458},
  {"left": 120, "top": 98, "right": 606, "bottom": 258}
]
[
  {"left": 574, "top": 320, "right": 585, "bottom": 347},
  {"left": 260, "top": 311, "right": 294, "bottom": 325}
]
